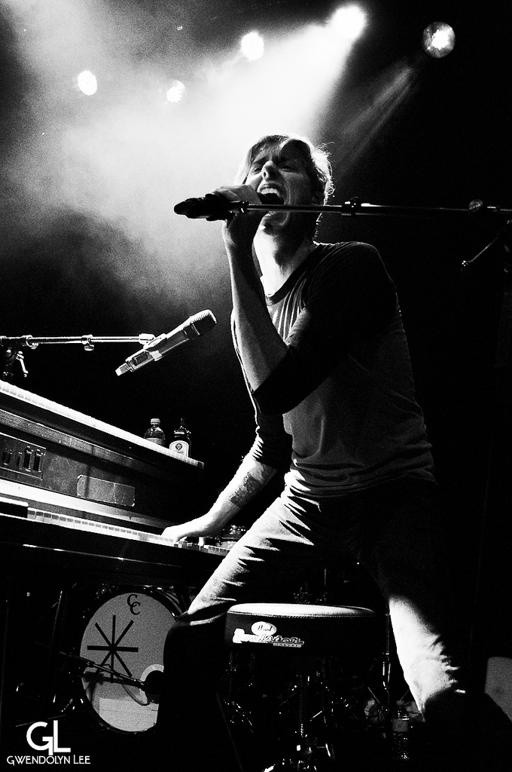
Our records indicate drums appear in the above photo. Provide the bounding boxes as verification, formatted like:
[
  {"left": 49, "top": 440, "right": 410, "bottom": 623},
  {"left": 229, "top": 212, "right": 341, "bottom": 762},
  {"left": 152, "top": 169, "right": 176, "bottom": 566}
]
[{"left": 63, "top": 574, "right": 197, "bottom": 747}]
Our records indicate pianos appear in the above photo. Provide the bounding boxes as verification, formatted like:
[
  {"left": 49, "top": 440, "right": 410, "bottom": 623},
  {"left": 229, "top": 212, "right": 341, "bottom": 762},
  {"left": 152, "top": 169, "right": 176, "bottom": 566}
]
[{"left": 2, "top": 378, "right": 234, "bottom": 572}]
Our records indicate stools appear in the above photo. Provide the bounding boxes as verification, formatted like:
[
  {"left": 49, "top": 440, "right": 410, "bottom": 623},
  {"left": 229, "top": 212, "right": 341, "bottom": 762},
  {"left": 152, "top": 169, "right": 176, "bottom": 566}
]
[{"left": 223, "top": 600, "right": 381, "bottom": 771}]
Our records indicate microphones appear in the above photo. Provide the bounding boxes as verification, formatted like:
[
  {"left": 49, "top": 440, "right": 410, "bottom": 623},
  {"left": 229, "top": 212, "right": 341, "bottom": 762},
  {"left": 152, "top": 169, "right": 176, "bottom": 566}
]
[
  {"left": 174, "top": 192, "right": 267, "bottom": 221},
  {"left": 116, "top": 309, "right": 218, "bottom": 376}
]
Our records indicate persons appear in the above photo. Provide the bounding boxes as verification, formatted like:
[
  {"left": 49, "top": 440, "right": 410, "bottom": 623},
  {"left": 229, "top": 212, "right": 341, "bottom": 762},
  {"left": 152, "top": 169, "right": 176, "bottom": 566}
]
[{"left": 158, "top": 132, "right": 512, "bottom": 771}]
